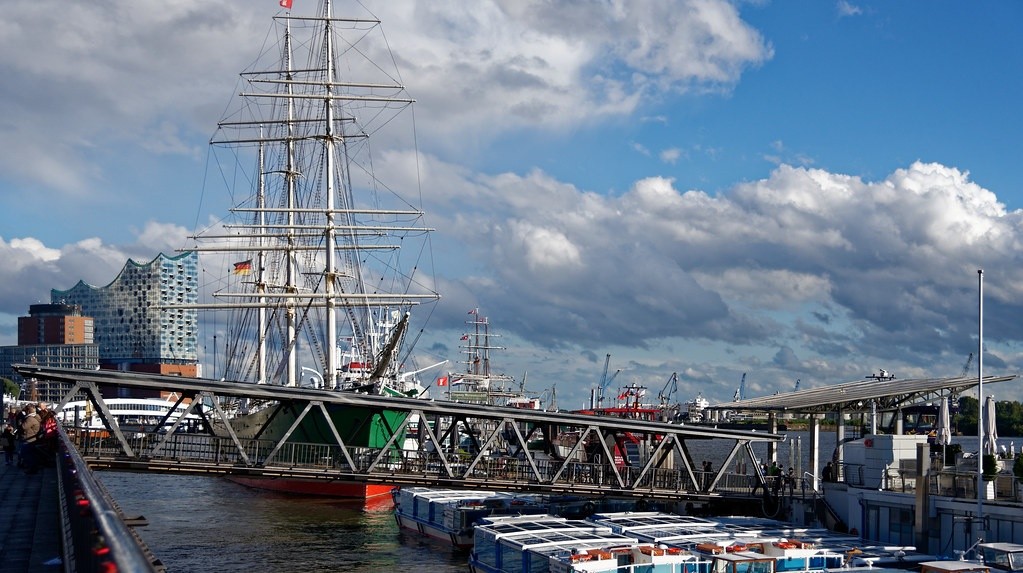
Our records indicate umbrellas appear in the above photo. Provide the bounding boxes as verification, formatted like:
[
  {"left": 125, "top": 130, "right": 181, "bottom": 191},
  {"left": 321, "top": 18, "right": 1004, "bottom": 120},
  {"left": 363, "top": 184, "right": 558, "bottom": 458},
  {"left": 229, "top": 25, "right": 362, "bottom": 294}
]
[
  {"left": 982, "top": 394, "right": 999, "bottom": 460},
  {"left": 934, "top": 396, "right": 953, "bottom": 470}
]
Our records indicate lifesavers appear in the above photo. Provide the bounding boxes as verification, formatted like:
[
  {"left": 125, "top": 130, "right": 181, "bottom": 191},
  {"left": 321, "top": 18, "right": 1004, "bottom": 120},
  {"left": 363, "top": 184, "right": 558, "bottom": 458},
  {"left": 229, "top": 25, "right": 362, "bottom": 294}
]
[
  {"left": 865, "top": 439, "right": 872, "bottom": 447},
  {"left": 777, "top": 542, "right": 796, "bottom": 548},
  {"left": 570, "top": 554, "right": 592, "bottom": 560},
  {"left": 726, "top": 546, "right": 747, "bottom": 551},
  {"left": 667, "top": 548, "right": 682, "bottom": 554}
]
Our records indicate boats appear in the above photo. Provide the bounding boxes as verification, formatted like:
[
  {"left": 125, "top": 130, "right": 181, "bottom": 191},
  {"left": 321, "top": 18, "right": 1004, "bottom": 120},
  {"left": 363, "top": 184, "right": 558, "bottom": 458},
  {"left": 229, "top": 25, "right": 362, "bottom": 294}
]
[
  {"left": 403, "top": 305, "right": 802, "bottom": 463},
  {"left": 390, "top": 480, "right": 1022, "bottom": 573},
  {"left": 0, "top": 405, "right": 166, "bottom": 573},
  {"left": 14, "top": 1, "right": 788, "bottom": 499},
  {"left": 703, "top": 268, "right": 1023, "bottom": 573}
]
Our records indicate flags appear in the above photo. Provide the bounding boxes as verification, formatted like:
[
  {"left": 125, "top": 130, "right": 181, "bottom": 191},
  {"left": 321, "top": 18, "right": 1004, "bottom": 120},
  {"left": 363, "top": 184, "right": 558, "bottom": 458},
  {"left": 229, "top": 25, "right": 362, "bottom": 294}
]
[
  {"left": 232, "top": 258, "right": 253, "bottom": 277},
  {"left": 460, "top": 335, "right": 468, "bottom": 341},
  {"left": 279, "top": 0, "right": 293, "bottom": 9},
  {"left": 437, "top": 376, "right": 449, "bottom": 387},
  {"left": 468, "top": 309, "right": 475, "bottom": 314}
]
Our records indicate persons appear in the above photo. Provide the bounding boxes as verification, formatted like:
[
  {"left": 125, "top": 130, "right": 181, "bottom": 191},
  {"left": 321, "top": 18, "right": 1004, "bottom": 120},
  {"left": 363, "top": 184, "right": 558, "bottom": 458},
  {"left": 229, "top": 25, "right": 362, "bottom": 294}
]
[
  {"left": 822, "top": 461, "right": 833, "bottom": 493},
  {"left": 764, "top": 461, "right": 785, "bottom": 496},
  {"left": 788, "top": 467, "right": 796, "bottom": 494},
  {"left": 2, "top": 400, "right": 59, "bottom": 472}
]
